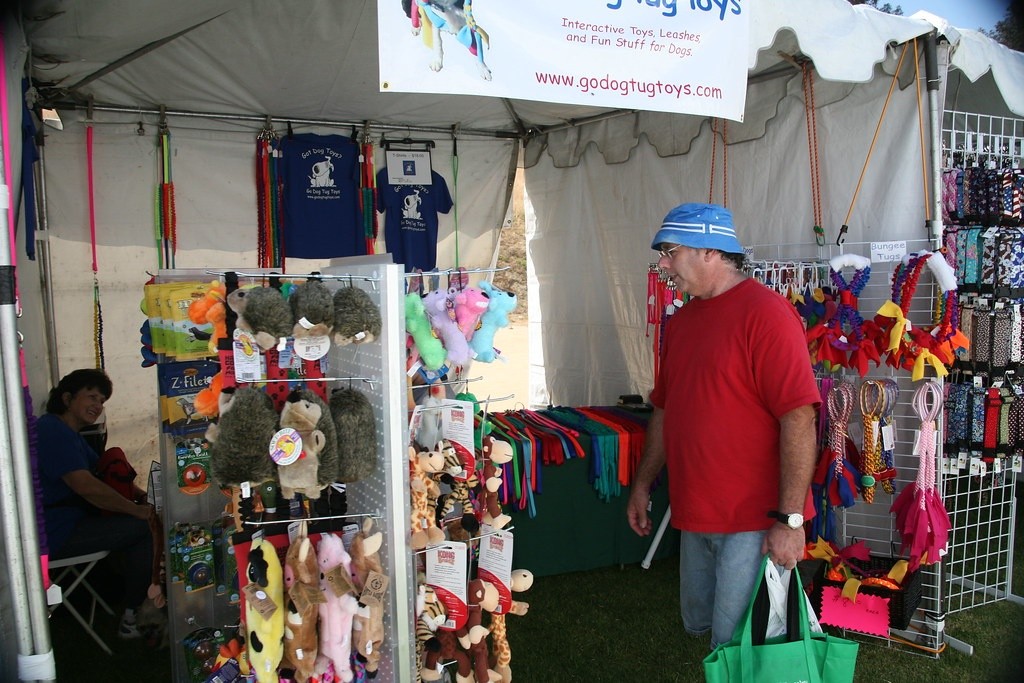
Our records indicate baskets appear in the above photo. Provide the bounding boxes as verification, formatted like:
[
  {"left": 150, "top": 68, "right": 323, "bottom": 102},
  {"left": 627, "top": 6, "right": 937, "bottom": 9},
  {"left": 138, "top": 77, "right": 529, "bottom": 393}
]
[{"left": 813, "top": 537, "right": 923, "bottom": 629}]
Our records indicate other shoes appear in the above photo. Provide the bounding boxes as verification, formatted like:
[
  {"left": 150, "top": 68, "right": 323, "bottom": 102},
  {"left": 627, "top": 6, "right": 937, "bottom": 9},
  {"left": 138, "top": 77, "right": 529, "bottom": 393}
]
[{"left": 118, "top": 619, "right": 147, "bottom": 638}]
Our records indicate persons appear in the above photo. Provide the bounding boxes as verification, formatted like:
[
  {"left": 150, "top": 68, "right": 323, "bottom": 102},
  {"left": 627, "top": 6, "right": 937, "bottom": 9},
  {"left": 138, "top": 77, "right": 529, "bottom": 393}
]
[
  {"left": 36, "top": 369, "right": 164, "bottom": 638},
  {"left": 626, "top": 202, "right": 823, "bottom": 654}
]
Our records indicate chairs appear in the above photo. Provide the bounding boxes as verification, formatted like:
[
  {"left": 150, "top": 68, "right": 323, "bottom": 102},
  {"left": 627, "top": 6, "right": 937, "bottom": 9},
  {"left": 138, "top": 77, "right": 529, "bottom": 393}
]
[{"left": 48, "top": 549, "right": 114, "bottom": 658}]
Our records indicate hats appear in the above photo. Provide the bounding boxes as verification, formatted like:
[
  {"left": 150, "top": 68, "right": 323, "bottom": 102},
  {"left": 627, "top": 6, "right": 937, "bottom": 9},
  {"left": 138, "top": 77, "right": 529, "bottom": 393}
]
[{"left": 651, "top": 203, "right": 746, "bottom": 256}]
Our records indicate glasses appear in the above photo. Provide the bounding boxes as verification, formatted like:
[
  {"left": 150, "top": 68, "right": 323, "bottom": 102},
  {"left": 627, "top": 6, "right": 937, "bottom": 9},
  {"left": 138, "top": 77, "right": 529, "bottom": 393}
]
[{"left": 658, "top": 245, "right": 681, "bottom": 261}]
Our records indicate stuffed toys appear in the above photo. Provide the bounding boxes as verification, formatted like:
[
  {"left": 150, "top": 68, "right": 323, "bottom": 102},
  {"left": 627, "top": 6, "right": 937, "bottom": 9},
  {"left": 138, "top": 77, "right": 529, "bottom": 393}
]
[
  {"left": 409, "top": 392, "right": 513, "bottom": 548},
  {"left": 216, "top": 516, "right": 384, "bottom": 683},
  {"left": 416, "top": 568, "right": 533, "bottom": 683},
  {"left": 208, "top": 385, "right": 378, "bottom": 529},
  {"left": 404, "top": 284, "right": 517, "bottom": 369},
  {"left": 190, "top": 271, "right": 381, "bottom": 417}
]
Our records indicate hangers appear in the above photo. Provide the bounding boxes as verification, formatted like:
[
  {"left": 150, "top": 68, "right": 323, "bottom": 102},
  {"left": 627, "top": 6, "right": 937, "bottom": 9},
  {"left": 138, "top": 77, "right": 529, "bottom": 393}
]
[{"left": 380, "top": 124, "right": 436, "bottom": 153}]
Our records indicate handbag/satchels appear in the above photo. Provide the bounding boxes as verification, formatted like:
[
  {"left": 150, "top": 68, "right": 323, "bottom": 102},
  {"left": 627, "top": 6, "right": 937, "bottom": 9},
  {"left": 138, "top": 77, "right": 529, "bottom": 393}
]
[
  {"left": 702, "top": 552, "right": 859, "bottom": 683},
  {"left": 95, "top": 446, "right": 136, "bottom": 502}
]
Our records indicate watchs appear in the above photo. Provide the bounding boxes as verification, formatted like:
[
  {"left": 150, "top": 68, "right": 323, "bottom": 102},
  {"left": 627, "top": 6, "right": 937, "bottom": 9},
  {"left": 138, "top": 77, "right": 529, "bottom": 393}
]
[{"left": 769, "top": 511, "right": 804, "bottom": 530}]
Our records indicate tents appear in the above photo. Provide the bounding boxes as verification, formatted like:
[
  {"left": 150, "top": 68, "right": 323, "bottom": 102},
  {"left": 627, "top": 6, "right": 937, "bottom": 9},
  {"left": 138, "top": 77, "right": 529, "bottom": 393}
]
[
  {"left": 926, "top": 18, "right": 1024, "bottom": 650},
  {"left": 0, "top": 0, "right": 942, "bottom": 683}
]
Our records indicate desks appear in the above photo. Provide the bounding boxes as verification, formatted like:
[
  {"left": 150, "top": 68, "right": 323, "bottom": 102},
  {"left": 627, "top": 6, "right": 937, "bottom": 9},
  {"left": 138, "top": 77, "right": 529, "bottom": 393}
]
[{"left": 503, "top": 405, "right": 674, "bottom": 577}]
